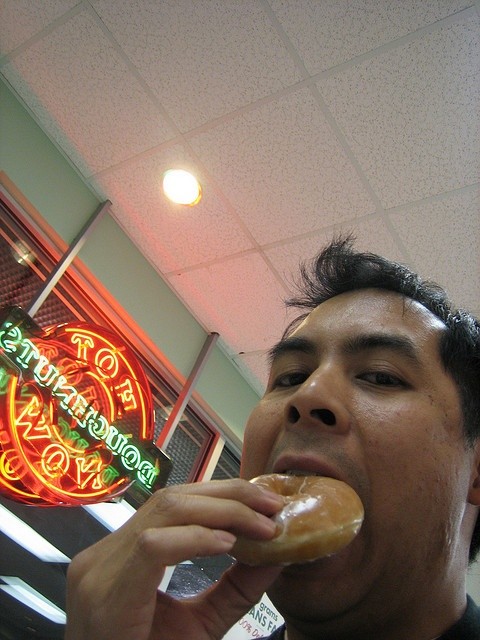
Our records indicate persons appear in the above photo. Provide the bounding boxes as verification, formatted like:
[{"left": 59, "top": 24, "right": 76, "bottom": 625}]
[{"left": 64, "top": 233, "right": 480, "bottom": 638}]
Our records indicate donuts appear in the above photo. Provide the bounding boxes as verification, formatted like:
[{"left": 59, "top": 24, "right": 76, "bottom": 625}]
[{"left": 227, "top": 473, "right": 365, "bottom": 565}]
